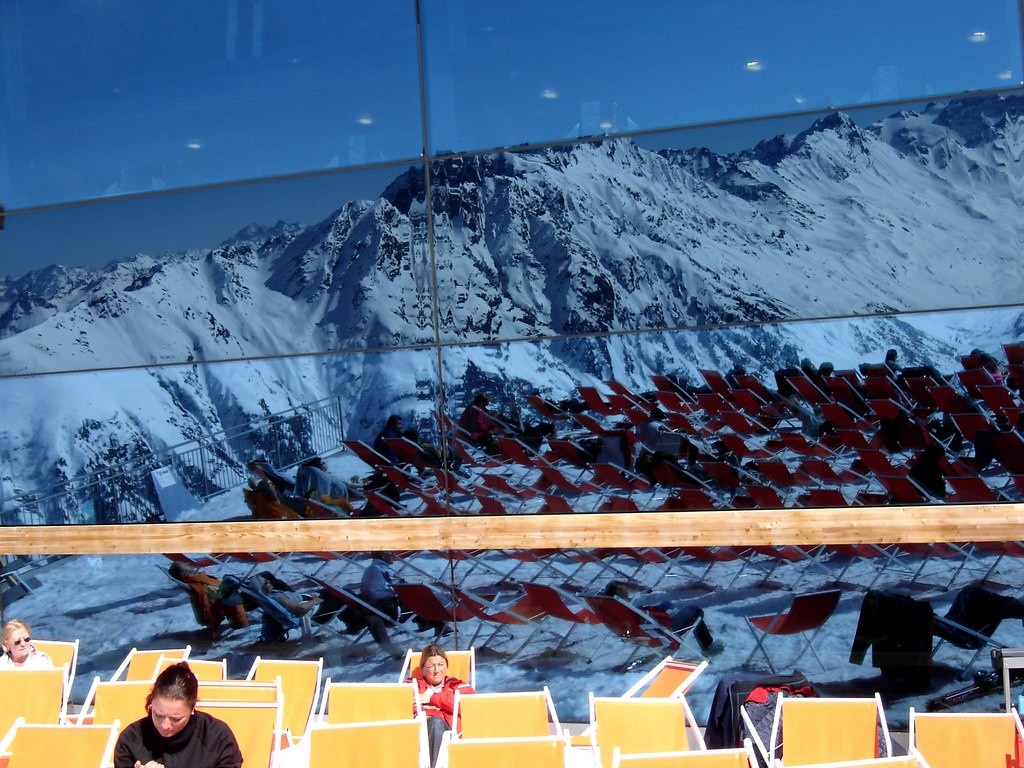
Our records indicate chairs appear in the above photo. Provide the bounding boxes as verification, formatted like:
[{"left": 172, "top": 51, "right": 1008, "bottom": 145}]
[{"left": 0, "top": 341, "right": 1024, "bottom": 768}]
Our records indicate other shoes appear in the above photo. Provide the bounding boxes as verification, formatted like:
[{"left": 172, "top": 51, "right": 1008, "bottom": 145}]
[
  {"left": 417, "top": 620, "right": 435, "bottom": 632},
  {"left": 434, "top": 625, "right": 459, "bottom": 636}
]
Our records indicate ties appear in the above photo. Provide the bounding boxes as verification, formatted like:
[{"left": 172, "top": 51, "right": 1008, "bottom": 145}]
[{"left": 386, "top": 571, "right": 393, "bottom": 582}]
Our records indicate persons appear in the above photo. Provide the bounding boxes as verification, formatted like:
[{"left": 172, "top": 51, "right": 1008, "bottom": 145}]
[
  {"left": 886, "top": 348, "right": 904, "bottom": 378},
  {"left": 734, "top": 363, "right": 764, "bottom": 380},
  {"left": 605, "top": 580, "right": 726, "bottom": 656},
  {"left": 0, "top": 619, "right": 56, "bottom": 669},
  {"left": 460, "top": 392, "right": 612, "bottom": 464},
  {"left": 374, "top": 415, "right": 467, "bottom": 476},
  {"left": 114, "top": 661, "right": 244, "bottom": 768},
  {"left": 666, "top": 372, "right": 712, "bottom": 403},
  {"left": 361, "top": 551, "right": 460, "bottom": 637},
  {"left": 801, "top": 358, "right": 835, "bottom": 398},
  {"left": 310, "top": 456, "right": 408, "bottom": 511},
  {"left": 403, "top": 644, "right": 477, "bottom": 768},
  {"left": 970, "top": 348, "right": 1009, "bottom": 386},
  {"left": 909, "top": 444, "right": 946, "bottom": 502},
  {"left": 649, "top": 406, "right": 760, "bottom": 482},
  {"left": 169, "top": 560, "right": 324, "bottom": 630}
]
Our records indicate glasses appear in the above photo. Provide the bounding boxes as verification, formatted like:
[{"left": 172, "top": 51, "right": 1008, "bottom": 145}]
[
  {"left": 320, "top": 461, "right": 326, "bottom": 465},
  {"left": 13, "top": 635, "right": 32, "bottom": 646},
  {"left": 264, "top": 579, "right": 269, "bottom": 586},
  {"left": 382, "top": 552, "right": 395, "bottom": 556}
]
